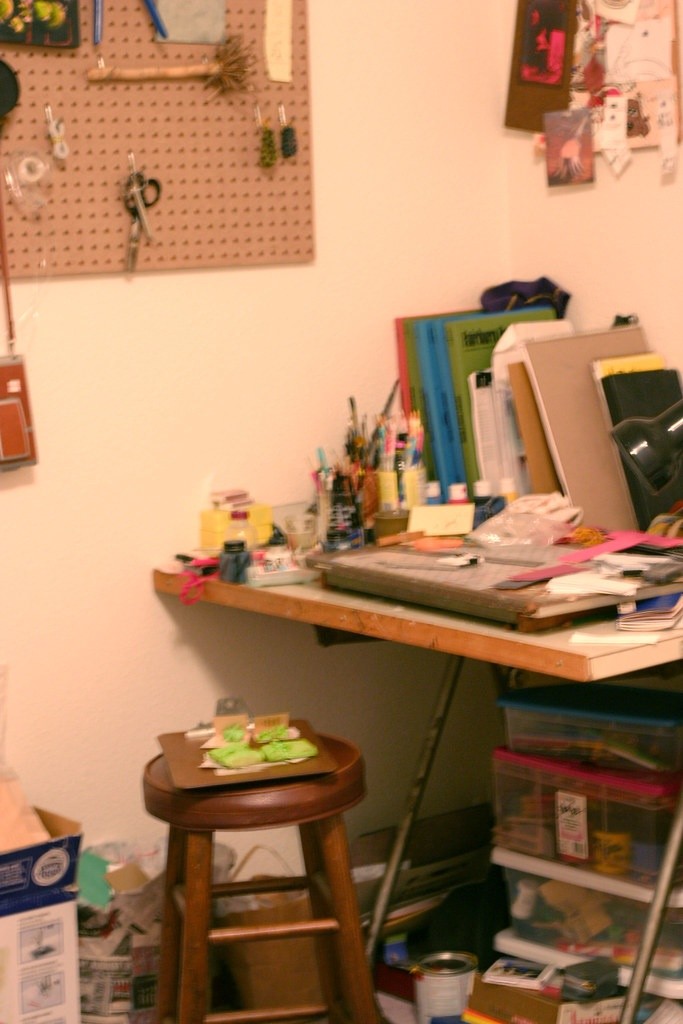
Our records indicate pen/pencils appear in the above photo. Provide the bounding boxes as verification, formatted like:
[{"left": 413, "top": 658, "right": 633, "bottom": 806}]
[{"left": 310, "top": 380, "right": 426, "bottom": 552}]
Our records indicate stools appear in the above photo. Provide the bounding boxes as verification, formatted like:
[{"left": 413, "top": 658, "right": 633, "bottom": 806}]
[{"left": 143, "top": 731, "right": 384, "bottom": 1024}]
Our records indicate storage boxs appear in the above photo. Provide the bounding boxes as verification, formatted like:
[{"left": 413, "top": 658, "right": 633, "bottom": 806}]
[
  {"left": 490, "top": 846, "right": 683, "bottom": 979},
  {"left": 493, "top": 682, "right": 683, "bottom": 775},
  {"left": 467, "top": 972, "right": 628, "bottom": 1024},
  {"left": 0, "top": 804, "right": 330, "bottom": 1024},
  {"left": 493, "top": 929, "right": 683, "bottom": 1024},
  {"left": 491, "top": 744, "right": 683, "bottom": 892}
]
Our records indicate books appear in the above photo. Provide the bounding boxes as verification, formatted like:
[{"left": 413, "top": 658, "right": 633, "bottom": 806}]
[{"left": 388, "top": 303, "right": 559, "bottom": 510}]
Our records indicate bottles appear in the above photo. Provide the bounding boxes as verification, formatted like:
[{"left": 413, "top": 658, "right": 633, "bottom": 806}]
[
  {"left": 470, "top": 478, "right": 504, "bottom": 526},
  {"left": 228, "top": 508, "right": 254, "bottom": 549},
  {"left": 425, "top": 479, "right": 441, "bottom": 504},
  {"left": 447, "top": 482, "right": 467, "bottom": 504},
  {"left": 219, "top": 537, "right": 252, "bottom": 583},
  {"left": 500, "top": 478, "right": 521, "bottom": 506}
]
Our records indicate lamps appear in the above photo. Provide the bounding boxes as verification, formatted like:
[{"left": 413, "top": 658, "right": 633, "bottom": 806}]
[{"left": 610, "top": 398, "right": 683, "bottom": 493}]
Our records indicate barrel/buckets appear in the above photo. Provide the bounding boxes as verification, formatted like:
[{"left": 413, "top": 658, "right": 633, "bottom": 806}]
[
  {"left": 379, "top": 469, "right": 400, "bottom": 513},
  {"left": 414, "top": 951, "right": 481, "bottom": 1024}
]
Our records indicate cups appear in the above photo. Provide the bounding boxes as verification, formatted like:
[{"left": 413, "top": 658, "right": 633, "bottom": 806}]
[{"left": 284, "top": 514, "right": 322, "bottom": 552}]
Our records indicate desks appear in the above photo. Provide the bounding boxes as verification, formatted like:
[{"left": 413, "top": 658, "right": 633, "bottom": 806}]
[{"left": 154, "top": 566, "right": 683, "bottom": 974}]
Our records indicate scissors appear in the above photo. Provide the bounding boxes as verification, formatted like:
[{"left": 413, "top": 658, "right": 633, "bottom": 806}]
[{"left": 122, "top": 171, "right": 162, "bottom": 273}]
